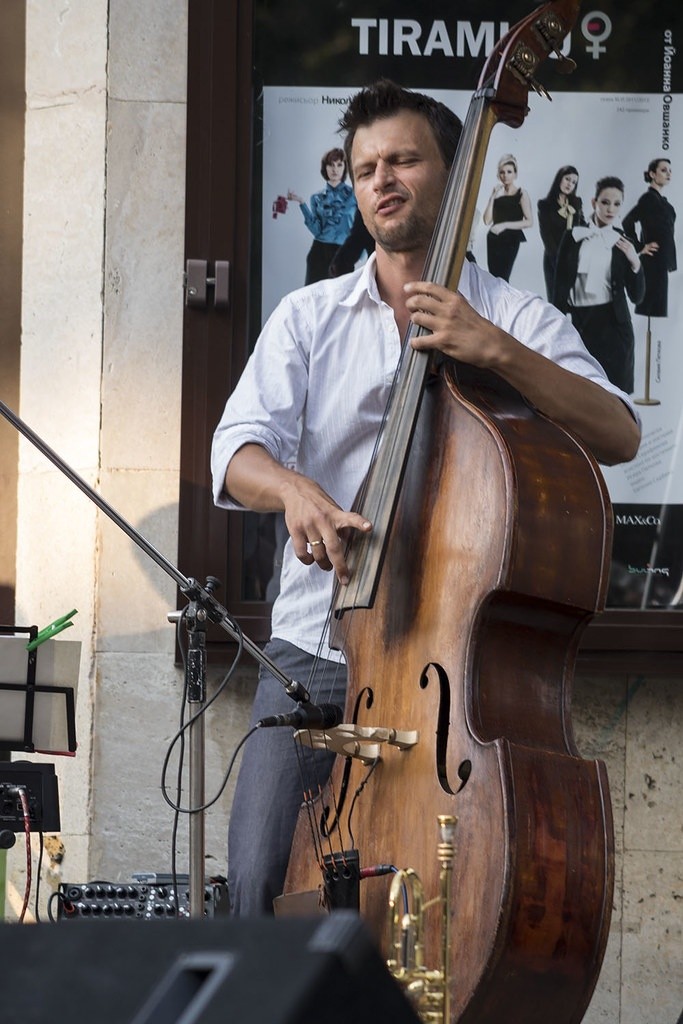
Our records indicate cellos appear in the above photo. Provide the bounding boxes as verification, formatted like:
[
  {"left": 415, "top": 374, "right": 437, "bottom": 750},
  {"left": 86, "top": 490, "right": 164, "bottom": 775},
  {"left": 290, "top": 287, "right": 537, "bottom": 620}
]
[{"left": 276, "top": 3, "right": 626, "bottom": 1024}]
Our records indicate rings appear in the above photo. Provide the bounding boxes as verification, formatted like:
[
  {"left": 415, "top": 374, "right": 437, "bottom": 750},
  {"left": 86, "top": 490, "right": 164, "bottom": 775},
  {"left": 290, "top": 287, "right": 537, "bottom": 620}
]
[{"left": 310, "top": 540, "right": 320, "bottom": 546}]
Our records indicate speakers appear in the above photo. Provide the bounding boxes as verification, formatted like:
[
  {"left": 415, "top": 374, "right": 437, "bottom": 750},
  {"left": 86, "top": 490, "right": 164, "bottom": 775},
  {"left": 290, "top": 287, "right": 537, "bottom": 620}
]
[{"left": 0, "top": 910, "right": 426, "bottom": 1024}]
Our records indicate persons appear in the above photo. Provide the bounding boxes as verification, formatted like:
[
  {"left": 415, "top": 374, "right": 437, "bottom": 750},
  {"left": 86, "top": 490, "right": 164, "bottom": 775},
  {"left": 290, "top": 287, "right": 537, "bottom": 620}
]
[
  {"left": 623, "top": 157, "right": 677, "bottom": 318},
  {"left": 277, "top": 148, "right": 357, "bottom": 288},
  {"left": 209, "top": 79, "right": 643, "bottom": 920},
  {"left": 537, "top": 165, "right": 589, "bottom": 312},
  {"left": 551, "top": 176, "right": 645, "bottom": 396},
  {"left": 483, "top": 153, "right": 533, "bottom": 284},
  {"left": 329, "top": 208, "right": 377, "bottom": 278}
]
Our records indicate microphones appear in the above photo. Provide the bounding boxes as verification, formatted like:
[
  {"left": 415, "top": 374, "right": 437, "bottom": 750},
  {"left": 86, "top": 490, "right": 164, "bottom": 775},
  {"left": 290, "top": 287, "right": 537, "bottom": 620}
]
[{"left": 258, "top": 704, "right": 344, "bottom": 729}]
